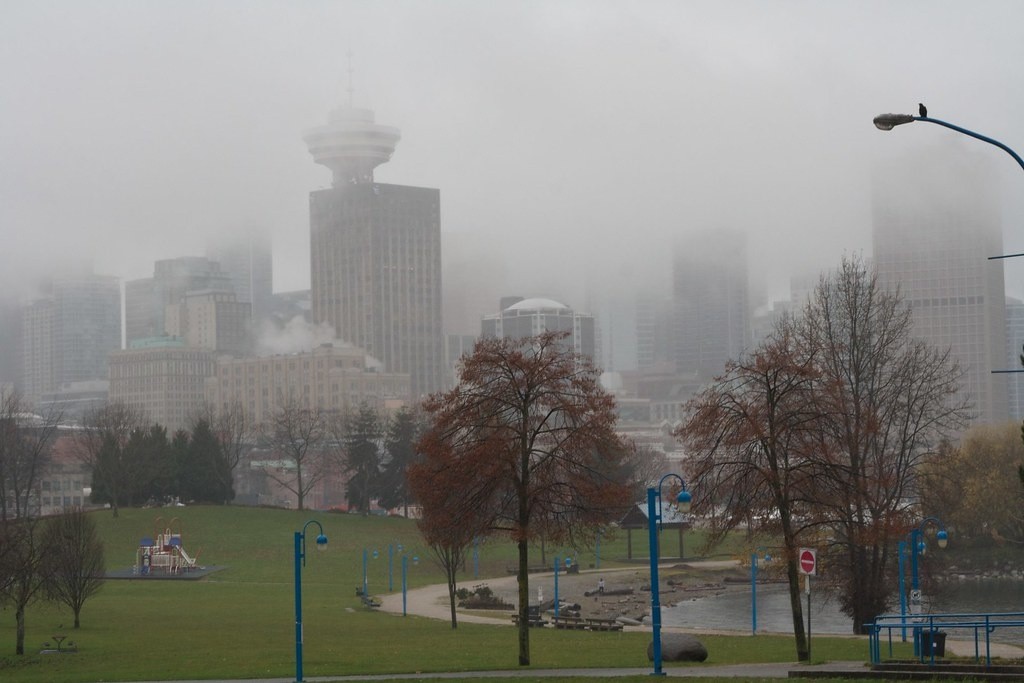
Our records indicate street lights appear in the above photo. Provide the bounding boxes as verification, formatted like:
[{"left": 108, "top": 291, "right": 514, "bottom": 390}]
[
  {"left": 294, "top": 519, "right": 328, "bottom": 682},
  {"left": 751, "top": 546, "right": 771, "bottom": 636},
  {"left": 363, "top": 547, "right": 379, "bottom": 599},
  {"left": 897, "top": 532, "right": 927, "bottom": 642},
  {"left": 647, "top": 474, "right": 693, "bottom": 676},
  {"left": 913, "top": 516, "right": 948, "bottom": 633},
  {"left": 389, "top": 544, "right": 403, "bottom": 592},
  {"left": 402, "top": 555, "right": 419, "bottom": 617},
  {"left": 554, "top": 557, "right": 572, "bottom": 623}
]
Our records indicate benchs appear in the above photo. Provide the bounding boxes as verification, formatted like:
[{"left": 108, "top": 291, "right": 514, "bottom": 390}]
[
  {"left": 585, "top": 618, "right": 624, "bottom": 631},
  {"left": 511, "top": 615, "right": 549, "bottom": 627},
  {"left": 551, "top": 617, "right": 588, "bottom": 629},
  {"left": 507, "top": 563, "right": 568, "bottom": 575}
]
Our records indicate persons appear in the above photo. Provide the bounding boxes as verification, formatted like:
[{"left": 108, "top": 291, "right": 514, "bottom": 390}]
[
  {"left": 599, "top": 578, "right": 604, "bottom": 593},
  {"left": 356, "top": 587, "right": 364, "bottom": 595}
]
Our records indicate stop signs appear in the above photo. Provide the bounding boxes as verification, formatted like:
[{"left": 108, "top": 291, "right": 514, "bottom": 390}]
[{"left": 798, "top": 549, "right": 818, "bottom": 574}]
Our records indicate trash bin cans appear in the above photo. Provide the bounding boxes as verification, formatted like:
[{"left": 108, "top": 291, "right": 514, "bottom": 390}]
[
  {"left": 921, "top": 630, "right": 948, "bottom": 656},
  {"left": 528, "top": 606, "right": 539, "bottom": 619}
]
